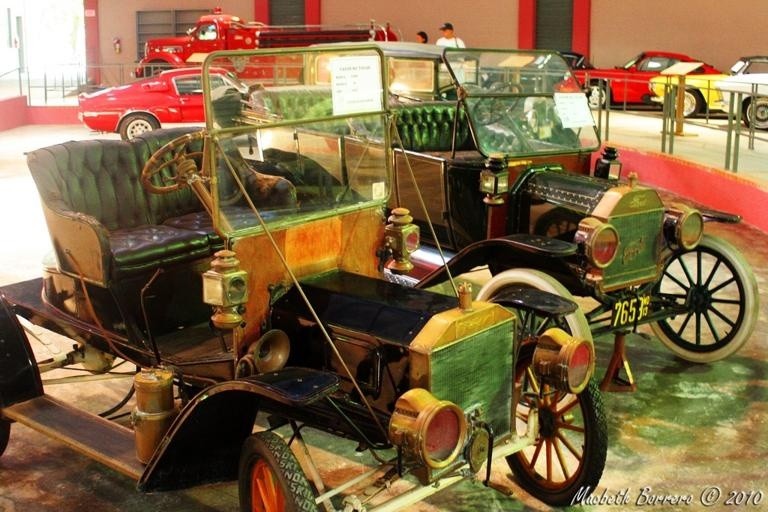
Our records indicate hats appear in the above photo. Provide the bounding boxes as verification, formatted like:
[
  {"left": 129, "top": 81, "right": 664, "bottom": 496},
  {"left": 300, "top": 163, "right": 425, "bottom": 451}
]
[{"left": 438, "top": 23, "right": 454, "bottom": 30}]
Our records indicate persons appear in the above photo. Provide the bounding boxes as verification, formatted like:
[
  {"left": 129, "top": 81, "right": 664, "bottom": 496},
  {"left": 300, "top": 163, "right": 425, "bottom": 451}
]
[
  {"left": 416, "top": 32, "right": 428, "bottom": 43},
  {"left": 437, "top": 23, "right": 465, "bottom": 48}
]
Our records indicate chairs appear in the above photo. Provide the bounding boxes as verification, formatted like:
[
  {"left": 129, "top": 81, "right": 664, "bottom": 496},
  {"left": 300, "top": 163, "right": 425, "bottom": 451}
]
[
  {"left": 250, "top": 84, "right": 470, "bottom": 151},
  {"left": 22, "top": 127, "right": 297, "bottom": 289}
]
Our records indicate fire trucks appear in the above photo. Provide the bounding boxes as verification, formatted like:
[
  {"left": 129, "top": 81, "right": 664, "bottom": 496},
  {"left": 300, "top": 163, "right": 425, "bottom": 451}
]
[{"left": 133, "top": 6, "right": 404, "bottom": 79}]
